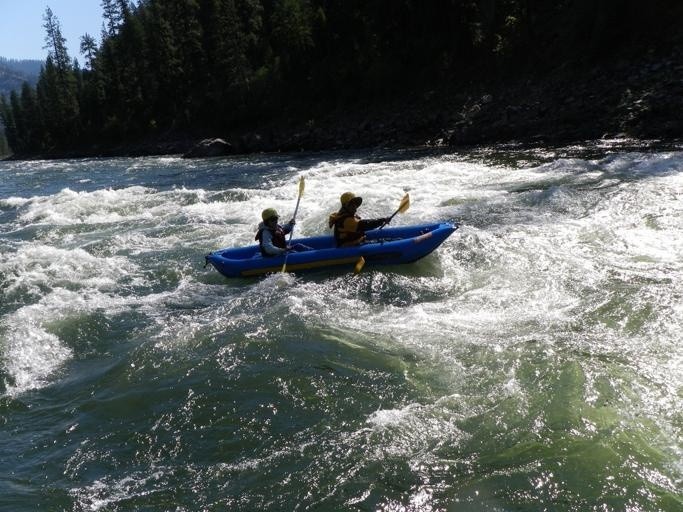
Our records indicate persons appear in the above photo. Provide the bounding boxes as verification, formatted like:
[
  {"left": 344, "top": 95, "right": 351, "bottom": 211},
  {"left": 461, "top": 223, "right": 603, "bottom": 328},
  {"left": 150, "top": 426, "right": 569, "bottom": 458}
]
[
  {"left": 329, "top": 192, "right": 404, "bottom": 248},
  {"left": 254, "top": 208, "right": 313, "bottom": 258}
]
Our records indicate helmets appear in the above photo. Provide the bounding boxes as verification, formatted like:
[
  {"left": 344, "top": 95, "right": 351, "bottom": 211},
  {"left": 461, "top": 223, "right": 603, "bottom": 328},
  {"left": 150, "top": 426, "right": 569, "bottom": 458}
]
[{"left": 262, "top": 208, "right": 279, "bottom": 221}]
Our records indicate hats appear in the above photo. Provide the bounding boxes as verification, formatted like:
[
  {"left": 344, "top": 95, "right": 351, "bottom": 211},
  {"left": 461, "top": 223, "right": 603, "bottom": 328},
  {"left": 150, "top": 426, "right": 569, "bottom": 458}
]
[{"left": 339, "top": 192, "right": 362, "bottom": 214}]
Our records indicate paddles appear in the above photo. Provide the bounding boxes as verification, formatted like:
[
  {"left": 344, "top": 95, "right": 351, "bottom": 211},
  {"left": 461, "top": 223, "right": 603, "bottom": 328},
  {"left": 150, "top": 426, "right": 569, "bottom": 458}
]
[
  {"left": 379, "top": 194, "right": 409, "bottom": 229},
  {"left": 282, "top": 175, "right": 305, "bottom": 273}
]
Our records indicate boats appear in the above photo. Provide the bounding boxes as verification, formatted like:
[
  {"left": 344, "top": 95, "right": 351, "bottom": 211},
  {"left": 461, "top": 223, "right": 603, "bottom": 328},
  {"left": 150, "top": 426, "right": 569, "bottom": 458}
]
[{"left": 203, "top": 221, "right": 460, "bottom": 279}]
